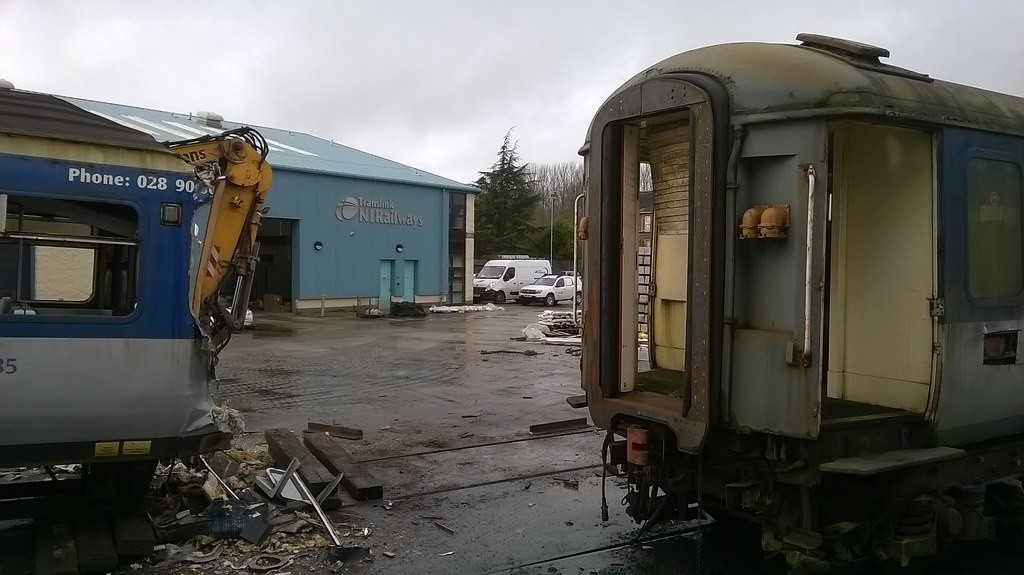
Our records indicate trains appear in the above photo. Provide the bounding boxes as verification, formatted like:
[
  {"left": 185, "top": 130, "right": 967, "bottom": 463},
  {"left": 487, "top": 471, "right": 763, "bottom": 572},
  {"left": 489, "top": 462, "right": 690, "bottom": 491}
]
[
  {"left": 0, "top": 80, "right": 245, "bottom": 495},
  {"left": 571, "top": 36, "right": 1024, "bottom": 556}
]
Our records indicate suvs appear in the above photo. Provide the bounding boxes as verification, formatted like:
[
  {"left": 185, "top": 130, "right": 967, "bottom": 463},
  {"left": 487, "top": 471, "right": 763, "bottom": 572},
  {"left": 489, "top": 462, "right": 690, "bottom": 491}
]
[{"left": 518, "top": 273, "right": 583, "bottom": 307}]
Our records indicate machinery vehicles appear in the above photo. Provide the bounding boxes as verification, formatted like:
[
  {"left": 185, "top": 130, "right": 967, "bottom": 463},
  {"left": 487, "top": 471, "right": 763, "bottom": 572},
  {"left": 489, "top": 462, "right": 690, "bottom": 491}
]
[{"left": 166, "top": 126, "right": 274, "bottom": 370}]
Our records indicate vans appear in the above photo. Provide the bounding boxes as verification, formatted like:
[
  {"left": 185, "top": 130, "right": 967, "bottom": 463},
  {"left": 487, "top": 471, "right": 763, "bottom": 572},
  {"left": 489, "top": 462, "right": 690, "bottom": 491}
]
[{"left": 474, "top": 260, "right": 554, "bottom": 305}]
